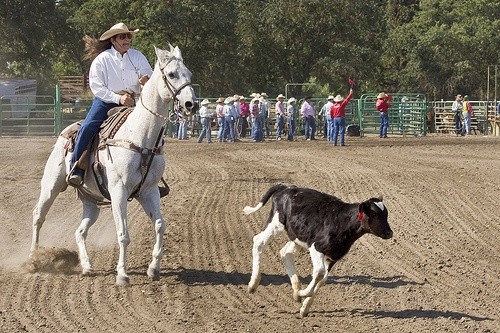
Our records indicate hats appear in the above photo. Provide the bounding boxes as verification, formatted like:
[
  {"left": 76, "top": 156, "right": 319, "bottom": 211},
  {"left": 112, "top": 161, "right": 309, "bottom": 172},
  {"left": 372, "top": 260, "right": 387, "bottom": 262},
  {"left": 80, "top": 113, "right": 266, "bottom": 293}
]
[
  {"left": 333, "top": 95, "right": 344, "bottom": 102},
  {"left": 456, "top": 95, "right": 462, "bottom": 100},
  {"left": 100, "top": 23, "right": 139, "bottom": 40},
  {"left": 288, "top": 97, "right": 297, "bottom": 104},
  {"left": 305, "top": 95, "right": 313, "bottom": 99},
  {"left": 377, "top": 93, "right": 388, "bottom": 99},
  {"left": 216, "top": 97, "right": 225, "bottom": 103},
  {"left": 233, "top": 95, "right": 240, "bottom": 100},
  {"left": 240, "top": 96, "right": 245, "bottom": 100},
  {"left": 201, "top": 100, "right": 211, "bottom": 106},
  {"left": 250, "top": 92, "right": 270, "bottom": 98},
  {"left": 224, "top": 97, "right": 234, "bottom": 103},
  {"left": 276, "top": 94, "right": 285, "bottom": 101},
  {"left": 328, "top": 96, "right": 335, "bottom": 100}
]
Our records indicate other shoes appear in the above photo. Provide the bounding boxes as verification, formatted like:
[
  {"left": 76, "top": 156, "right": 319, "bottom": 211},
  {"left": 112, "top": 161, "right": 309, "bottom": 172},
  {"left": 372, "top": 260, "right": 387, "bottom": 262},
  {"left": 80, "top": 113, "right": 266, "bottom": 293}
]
[
  {"left": 66, "top": 173, "right": 83, "bottom": 185},
  {"left": 379, "top": 135, "right": 388, "bottom": 138},
  {"left": 332, "top": 143, "right": 337, "bottom": 146},
  {"left": 340, "top": 144, "right": 346, "bottom": 146},
  {"left": 159, "top": 185, "right": 169, "bottom": 198}
]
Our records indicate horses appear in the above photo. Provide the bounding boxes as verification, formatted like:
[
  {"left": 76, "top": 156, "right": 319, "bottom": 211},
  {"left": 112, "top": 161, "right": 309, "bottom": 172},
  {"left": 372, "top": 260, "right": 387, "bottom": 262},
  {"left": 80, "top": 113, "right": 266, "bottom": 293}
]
[{"left": 19, "top": 39, "right": 200, "bottom": 286}]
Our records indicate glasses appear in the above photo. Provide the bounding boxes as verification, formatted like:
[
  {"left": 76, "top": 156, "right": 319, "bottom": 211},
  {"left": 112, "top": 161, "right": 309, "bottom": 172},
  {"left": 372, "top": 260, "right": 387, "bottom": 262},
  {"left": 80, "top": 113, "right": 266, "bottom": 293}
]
[{"left": 116, "top": 34, "right": 133, "bottom": 40}]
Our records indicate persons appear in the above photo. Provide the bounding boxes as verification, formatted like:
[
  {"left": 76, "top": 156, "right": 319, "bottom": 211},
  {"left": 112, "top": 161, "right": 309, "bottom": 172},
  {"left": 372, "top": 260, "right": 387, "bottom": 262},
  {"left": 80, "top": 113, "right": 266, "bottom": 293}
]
[
  {"left": 216, "top": 93, "right": 271, "bottom": 144},
  {"left": 330, "top": 89, "right": 353, "bottom": 146},
  {"left": 321, "top": 95, "right": 334, "bottom": 142},
  {"left": 275, "top": 94, "right": 286, "bottom": 141},
  {"left": 287, "top": 96, "right": 297, "bottom": 141},
  {"left": 300, "top": 95, "right": 317, "bottom": 142},
  {"left": 66, "top": 22, "right": 168, "bottom": 197},
  {"left": 399, "top": 96, "right": 410, "bottom": 133},
  {"left": 197, "top": 99, "right": 213, "bottom": 143},
  {"left": 375, "top": 92, "right": 393, "bottom": 138},
  {"left": 451, "top": 94, "right": 463, "bottom": 137},
  {"left": 415, "top": 95, "right": 425, "bottom": 133},
  {"left": 462, "top": 95, "right": 473, "bottom": 135},
  {"left": 177, "top": 119, "right": 189, "bottom": 140}
]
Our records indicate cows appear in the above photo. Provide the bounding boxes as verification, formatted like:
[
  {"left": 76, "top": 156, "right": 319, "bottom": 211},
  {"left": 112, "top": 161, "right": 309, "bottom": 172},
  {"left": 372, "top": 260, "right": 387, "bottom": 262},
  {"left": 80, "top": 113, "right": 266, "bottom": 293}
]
[{"left": 235, "top": 182, "right": 396, "bottom": 319}]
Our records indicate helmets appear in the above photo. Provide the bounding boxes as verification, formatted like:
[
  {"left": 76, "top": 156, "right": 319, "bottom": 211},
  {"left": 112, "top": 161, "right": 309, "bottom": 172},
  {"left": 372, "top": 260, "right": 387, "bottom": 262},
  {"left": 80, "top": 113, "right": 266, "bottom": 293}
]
[{"left": 464, "top": 95, "right": 470, "bottom": 99}]
